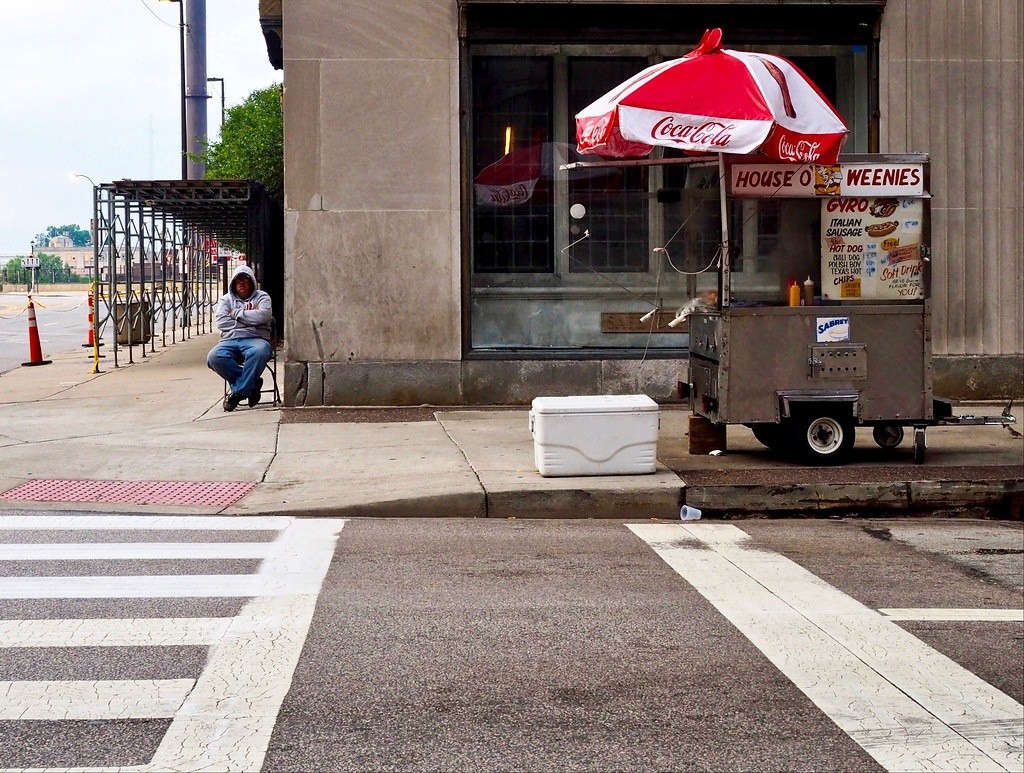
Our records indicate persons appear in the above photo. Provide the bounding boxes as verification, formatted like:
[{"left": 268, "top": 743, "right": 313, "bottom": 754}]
[{"left": 206, "top": 266, "right": 272, "bottom": 412}]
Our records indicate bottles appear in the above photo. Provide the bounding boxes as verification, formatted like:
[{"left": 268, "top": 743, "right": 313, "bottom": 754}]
[
  {"left": 790, "top": 281, "right": 800, "bottom": 306},
  {"left": 804, "top": 276, "right": 814, "bottom": 306}
]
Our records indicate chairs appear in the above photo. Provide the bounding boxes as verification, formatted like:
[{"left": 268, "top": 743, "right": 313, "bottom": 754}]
[{"left": 224, "top": 317, "right": 282, "bottom": 412}]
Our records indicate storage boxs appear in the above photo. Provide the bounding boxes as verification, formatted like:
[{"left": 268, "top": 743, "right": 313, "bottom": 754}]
[{"left": 528, "top": 394, "right": 661, "bottom": 477}]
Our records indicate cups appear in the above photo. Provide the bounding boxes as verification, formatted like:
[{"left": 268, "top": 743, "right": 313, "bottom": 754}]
[{"left": 680, "top": 505, "right": 702, "bottom": 521}]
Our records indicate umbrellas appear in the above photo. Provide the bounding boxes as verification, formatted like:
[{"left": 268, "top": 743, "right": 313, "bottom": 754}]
[
  {"left": 473, "top": 137, "right": 620, "bottom": 252},
  {"left": 575, "top": 29, "right": 850, "bottom": 309}
]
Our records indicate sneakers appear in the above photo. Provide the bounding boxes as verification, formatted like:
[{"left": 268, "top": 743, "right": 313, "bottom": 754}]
[
  {"left": 248, "top": 378, "right": 263, "bottom": 407},
  {"left": 223, "top": 393, "right": 241, "bottom": 411}
]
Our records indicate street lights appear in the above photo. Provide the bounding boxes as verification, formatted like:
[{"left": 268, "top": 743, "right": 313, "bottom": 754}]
[
  {"left": 73, "top": 172, "right": 104, "bottom": 281},
  {"left": 30, "top": 241, "right": 36, "bottom": 293}
]
